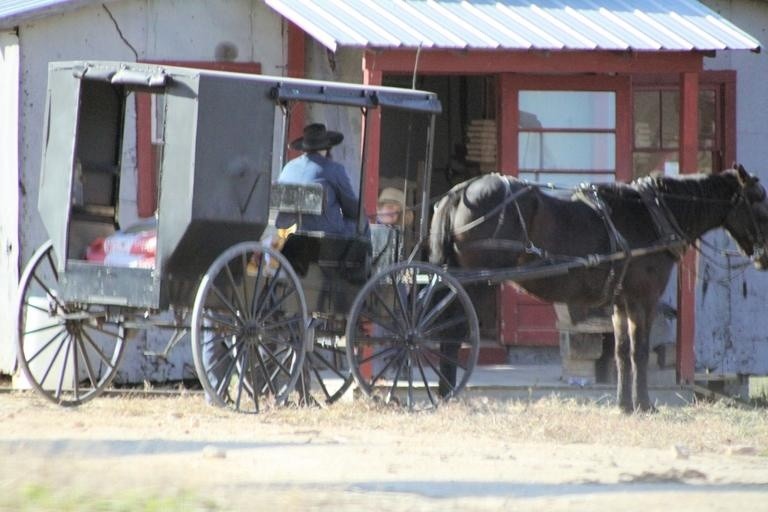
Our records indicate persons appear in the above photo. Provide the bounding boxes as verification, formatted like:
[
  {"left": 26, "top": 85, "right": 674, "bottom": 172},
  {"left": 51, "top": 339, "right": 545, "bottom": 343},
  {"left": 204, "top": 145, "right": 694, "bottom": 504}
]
[{"left": 275, "top": 141, "right": 368, "bottom": 244}]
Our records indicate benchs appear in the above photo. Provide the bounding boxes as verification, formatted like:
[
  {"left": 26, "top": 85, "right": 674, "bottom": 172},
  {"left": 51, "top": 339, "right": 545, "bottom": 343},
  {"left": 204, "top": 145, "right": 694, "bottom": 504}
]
[{"left": 270, "top": 181, "right": 359, "bottom": 266}]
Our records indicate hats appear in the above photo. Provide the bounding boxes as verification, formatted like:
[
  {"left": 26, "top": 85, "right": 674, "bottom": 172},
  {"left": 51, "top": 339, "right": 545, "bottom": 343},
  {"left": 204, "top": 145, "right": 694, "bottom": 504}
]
[{"left": 284, "top": 122, "right": 345, "bottom": 153}]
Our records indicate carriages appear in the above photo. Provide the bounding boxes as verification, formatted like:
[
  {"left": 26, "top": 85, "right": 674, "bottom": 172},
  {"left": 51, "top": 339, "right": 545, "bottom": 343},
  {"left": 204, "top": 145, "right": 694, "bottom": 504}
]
[{"left": 14, "top": 61, "right": 767, "bottom": 414}]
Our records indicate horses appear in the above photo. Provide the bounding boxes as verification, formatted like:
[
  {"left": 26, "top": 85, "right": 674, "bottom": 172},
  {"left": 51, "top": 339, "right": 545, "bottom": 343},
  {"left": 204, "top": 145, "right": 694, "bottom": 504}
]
[{"left": 426, "top": 160, "right": 768, "bottom": 415}]
